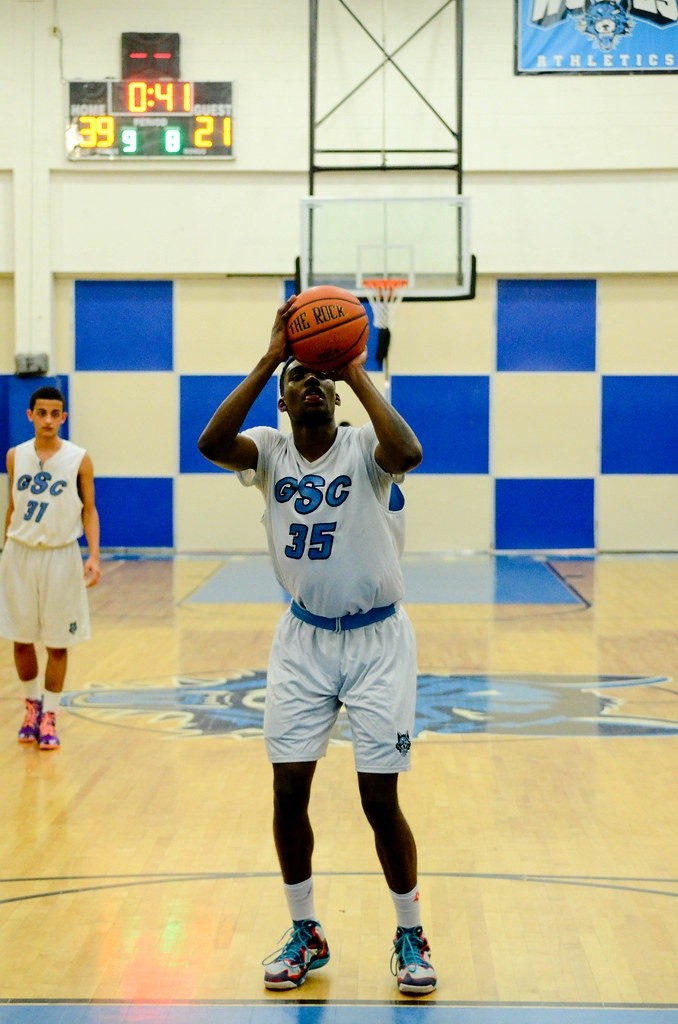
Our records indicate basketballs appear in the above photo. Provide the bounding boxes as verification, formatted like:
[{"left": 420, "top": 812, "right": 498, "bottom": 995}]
[{"left": 284, "top": 283, "right": 370, "bottom": 372}]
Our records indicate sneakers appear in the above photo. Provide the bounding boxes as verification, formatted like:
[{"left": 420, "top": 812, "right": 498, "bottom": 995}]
[
  {"left": 262, "top": 919, "right": 331, "bottom": 989},
  {"left": 390, "top": 926, "right": 438, "bottom": 993},
  {"left": 38, "top": 712, "right": 64, "bottom": 749},
  {"left": 17, "top": 698, "right": 43, "bottom": 743}
]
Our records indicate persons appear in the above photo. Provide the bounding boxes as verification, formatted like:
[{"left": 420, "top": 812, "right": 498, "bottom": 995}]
[
  {"left": 197, "top": 295, "right": 437, "bottom": 995},
  {"left": 0, "top": 386, "right": 102, "bottom": 750}
]
